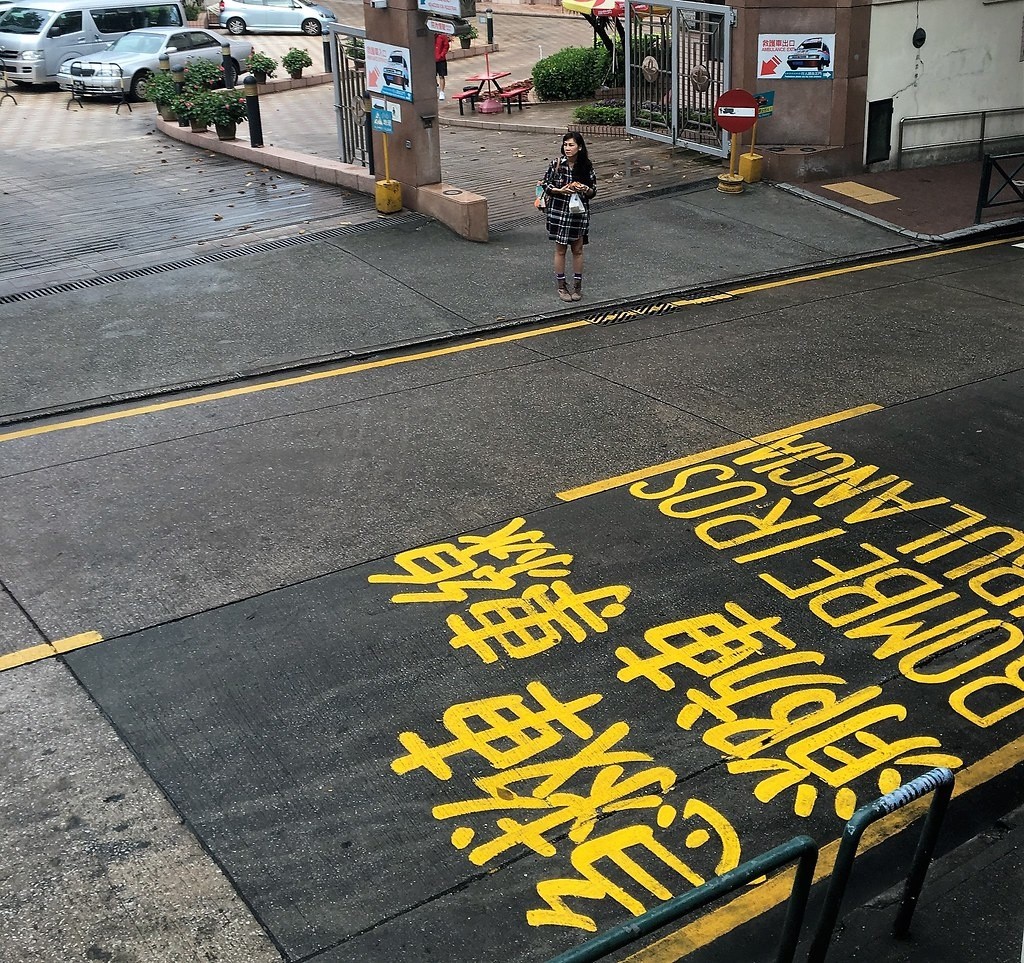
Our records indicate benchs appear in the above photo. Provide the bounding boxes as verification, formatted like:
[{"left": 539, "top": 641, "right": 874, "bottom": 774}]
[{"left": 452, "top": 90, "right": 478, "bottom": 116}]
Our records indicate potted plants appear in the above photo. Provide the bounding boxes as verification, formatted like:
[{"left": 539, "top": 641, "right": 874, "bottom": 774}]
[{"left": 345, "top": 40, "right": 365, "bottom": 68}]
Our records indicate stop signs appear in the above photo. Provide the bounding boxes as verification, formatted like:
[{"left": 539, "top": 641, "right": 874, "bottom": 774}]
[{"left": 712, "top": 87, "right": 759, "bottom": 134}]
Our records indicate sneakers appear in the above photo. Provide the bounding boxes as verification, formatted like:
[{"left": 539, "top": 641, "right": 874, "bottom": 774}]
[{"left": 438, "top": 90, "right": 446, "bottom": 100}]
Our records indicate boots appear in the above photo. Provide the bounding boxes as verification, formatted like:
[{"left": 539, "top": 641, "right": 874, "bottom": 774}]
[
  {"left": 571, "top": 274, "right": 582, "bottom": 301},
  {"left": 556, "top": 274, "right": 572, "bottom": 302}
]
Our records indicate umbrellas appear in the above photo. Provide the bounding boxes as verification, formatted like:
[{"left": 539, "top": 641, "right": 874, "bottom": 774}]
[{"left": 561, "top": 0, "right": 673, "bottom": 86}]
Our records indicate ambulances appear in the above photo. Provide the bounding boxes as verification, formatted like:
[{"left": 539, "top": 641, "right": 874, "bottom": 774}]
[
  {"left": 786, "top": 36, "right": 830, "bottom": 71},
  {"left": 383, "top": 48, "right": 409, "bottom": 90},
  {"left": 755, "top": 96, "right": 769, "bottom": 106}
]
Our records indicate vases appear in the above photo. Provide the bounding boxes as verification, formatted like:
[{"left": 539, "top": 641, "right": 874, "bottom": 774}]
[
  {"left": 291, "top": 69, "right": 302, "bottom": 80},
  {"left": 190, "top": 118, "right": 207, "bottom": 133},
  {"left": 156, "top": 102, "right": 164, "bottom": 115},
  {"left": 178, "top": 116, "right": 190, "bottom": 127},
  {"left": 460, "top": 39, "right": 471, "bottom": 49},
  {"left": 214, "top": 122, "right": 236, "bottom": 140},
  {"left": 159, "top": 105, "right": 178, "bottom": 121},
  {"left": 254, "top": 73, "right": 267, "bottom": 85}
]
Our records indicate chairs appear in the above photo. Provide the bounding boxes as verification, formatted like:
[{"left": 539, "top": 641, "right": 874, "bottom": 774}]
[{"left": 499, "top": 88, "right": 529, "bottom": 114}]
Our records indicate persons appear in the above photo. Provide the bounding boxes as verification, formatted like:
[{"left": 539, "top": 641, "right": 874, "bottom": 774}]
[
  {"left": 435, "top": 33, "right": 449, "bottom": 99},
  {"left": 542, "top": 131, "right": 597, "bottom": 302}
]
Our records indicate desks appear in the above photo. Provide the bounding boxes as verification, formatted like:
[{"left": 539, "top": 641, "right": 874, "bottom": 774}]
[{"left": 465, "top": 71, "right": 511, "bottom": 113}]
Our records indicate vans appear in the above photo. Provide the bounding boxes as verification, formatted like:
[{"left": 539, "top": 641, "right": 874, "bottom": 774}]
[{"left": 0, "top": 3, "right": 190, "bottom": 91}]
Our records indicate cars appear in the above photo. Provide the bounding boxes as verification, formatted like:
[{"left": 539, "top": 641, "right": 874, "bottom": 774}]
[
  {"left": 218, "top": 0, "right": 339, "bottom": 36},
  {"left": 53, "top": 26, "right": 255, "bottom": 98}
]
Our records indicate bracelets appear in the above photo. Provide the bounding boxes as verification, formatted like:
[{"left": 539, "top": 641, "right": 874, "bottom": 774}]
[{"left": 584, "top": 186, "right": 590, "bottom": 193}]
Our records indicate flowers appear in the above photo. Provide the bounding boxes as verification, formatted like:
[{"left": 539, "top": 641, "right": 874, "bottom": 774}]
[
  {"left": 459, "top": 27, "right": 479, "bottom": 40},
  {"left": 143, "top": 56, "right": 251, "bottom": 126},
  {"left": 245, "top": 51, "right": 278, "bottom": 79},
  {"left": 280, "top": 47, "right": 313, "bottom": 74}
]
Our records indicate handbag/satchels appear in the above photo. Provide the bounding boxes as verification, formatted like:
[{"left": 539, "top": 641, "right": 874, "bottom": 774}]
[{"left": 534, "top": 157, "right": 560, "bottom": 213}]
